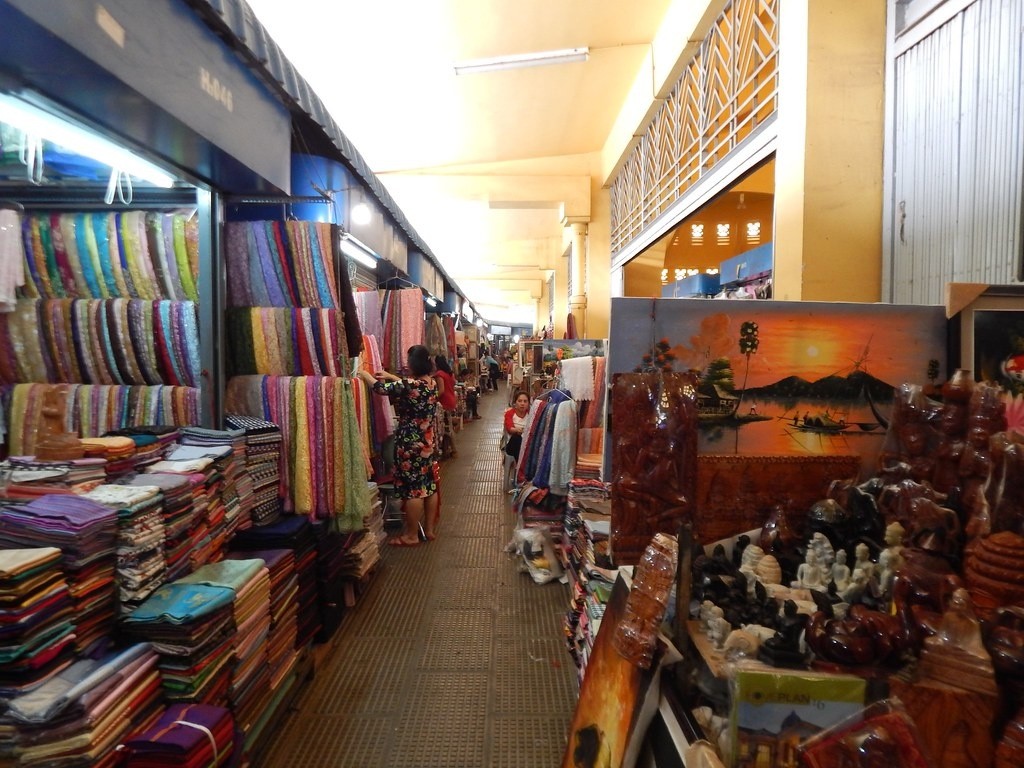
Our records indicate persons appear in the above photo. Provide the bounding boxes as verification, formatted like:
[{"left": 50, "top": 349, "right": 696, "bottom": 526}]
[
  {"left": 504, "top": 391, "right": 532, "bottom": 464},
  {"left": 356, "top": 345, "right": 438, "bottom": 546},
  {"left": 484, "top": 347, "right": 513, "bottom": 393},
  {"left": 456, "top": 368, "right": 482, "bottom": 419},
  {"left": 430, "top": 354, "right": 459, "bottom": 458},
  {"left": 790, "top": 522, "right": 993, "bottom": 666}
]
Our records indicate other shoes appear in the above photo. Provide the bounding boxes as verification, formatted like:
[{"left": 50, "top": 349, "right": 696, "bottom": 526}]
[
  {"left": 473, "top": 415, "right": 481, "bottom": 419},
  {"left": 450, "top": 448, "right": 458, "bottom": 456}
]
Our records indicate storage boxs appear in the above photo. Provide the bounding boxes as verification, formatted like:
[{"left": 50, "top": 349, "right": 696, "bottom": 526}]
[{"left": 728, "top": 666, "right": 866, "bottom": 768}]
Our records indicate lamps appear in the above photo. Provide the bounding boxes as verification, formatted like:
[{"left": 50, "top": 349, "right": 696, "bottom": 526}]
[{"left": 454, "top": 47, "right": 589, "bottom": 75}]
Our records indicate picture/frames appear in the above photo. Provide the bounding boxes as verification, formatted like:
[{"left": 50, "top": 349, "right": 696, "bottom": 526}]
[{"left": 960, "top": 285, "right": 1024, "bottom": 399}]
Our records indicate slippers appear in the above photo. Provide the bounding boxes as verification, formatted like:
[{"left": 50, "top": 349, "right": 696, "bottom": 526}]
[
  {"left": 388, "top": 536, "right": 418, "bottom": 547},
  {"left": 419, "top": 530, "right": 436, "bottom": 540}
]
